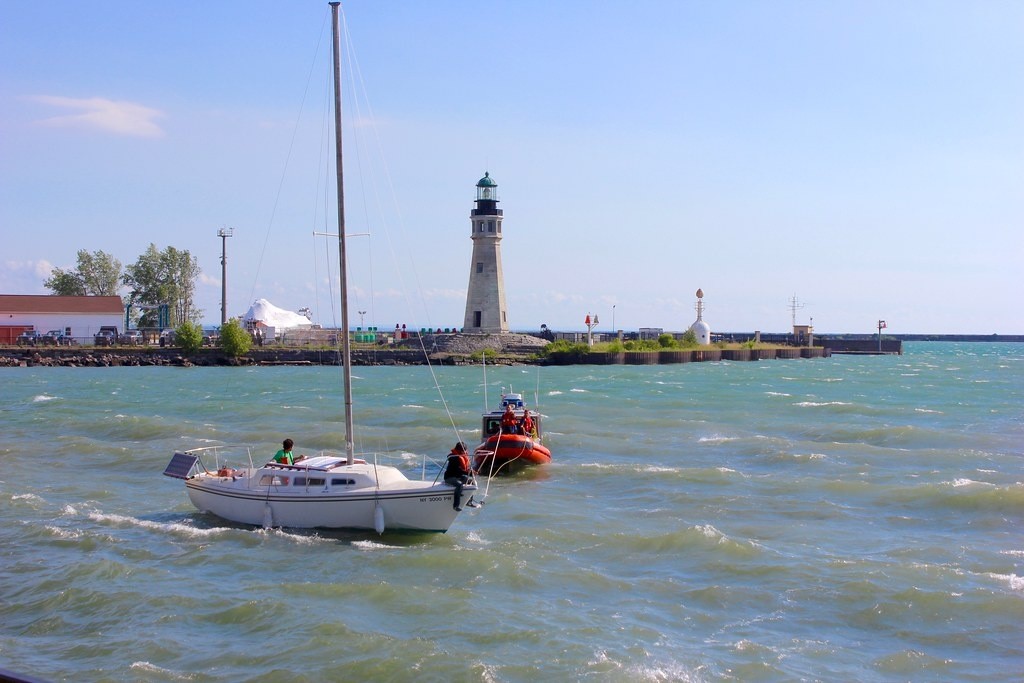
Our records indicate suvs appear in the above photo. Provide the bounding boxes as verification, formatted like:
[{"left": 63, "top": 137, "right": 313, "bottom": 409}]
[
  {"left": 200, "top": 330, "right": 211, "bottom": 347},
  {"left": 160, "top": 329, "right": 177, "bottom": 346}
]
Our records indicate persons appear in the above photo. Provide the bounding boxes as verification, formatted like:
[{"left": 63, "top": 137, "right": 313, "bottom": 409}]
[
  {"left": 270, "top": 438, "right": 305, "bottom": 486},
  {"left": 487, "top": 422, "right": 500, "bottom": 434},
  {"left": 520, "top": 409, "right": 535, "bottom": 437},
  {"left": 500, "top": 404, "right": 517, "bottom": 434},
  {"left": 444, "top": 442, "right": 478, "bottom": 512}
]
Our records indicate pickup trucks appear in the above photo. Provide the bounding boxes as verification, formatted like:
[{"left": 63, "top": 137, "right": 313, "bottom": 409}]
[
  {"left": 93, "top": 331, "right": 120, "bottom": 346},
  {"left": 41, "top": 330, "right": 73, "bottom": 346},
  {"left": 16, "top": 331, "right": 42, "bottom": 348},
  {"left": 119, "top": 330, "right": 151, "bottom": 347}
]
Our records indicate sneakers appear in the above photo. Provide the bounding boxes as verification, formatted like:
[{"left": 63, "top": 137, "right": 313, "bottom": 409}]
[
  {"left": 453, "top": 506, "right": 463, "bottom": 512},
  {"left": 466, "top": 502, "right": 476, "bottom": 507}
]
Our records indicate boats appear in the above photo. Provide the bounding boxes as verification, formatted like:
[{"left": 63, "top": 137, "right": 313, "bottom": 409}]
[{"left": 471, "top": 388, "right": 553, "bottom": 474}]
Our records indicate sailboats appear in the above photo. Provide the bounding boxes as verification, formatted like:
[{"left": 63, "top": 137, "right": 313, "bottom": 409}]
[{"left": 163, "top": 0, "right": 473, "bottom": 534}]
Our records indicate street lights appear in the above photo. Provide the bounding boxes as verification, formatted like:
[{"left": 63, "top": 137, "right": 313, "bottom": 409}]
[
  {"left": 212, "top": 326, "right": 217, "bottom": 345},
  {"left": 358, "top": 311, "right": 367, "bottom": 334},
  {"left": 613, "top": 305, "right": 615, "bottom": 333}
]
